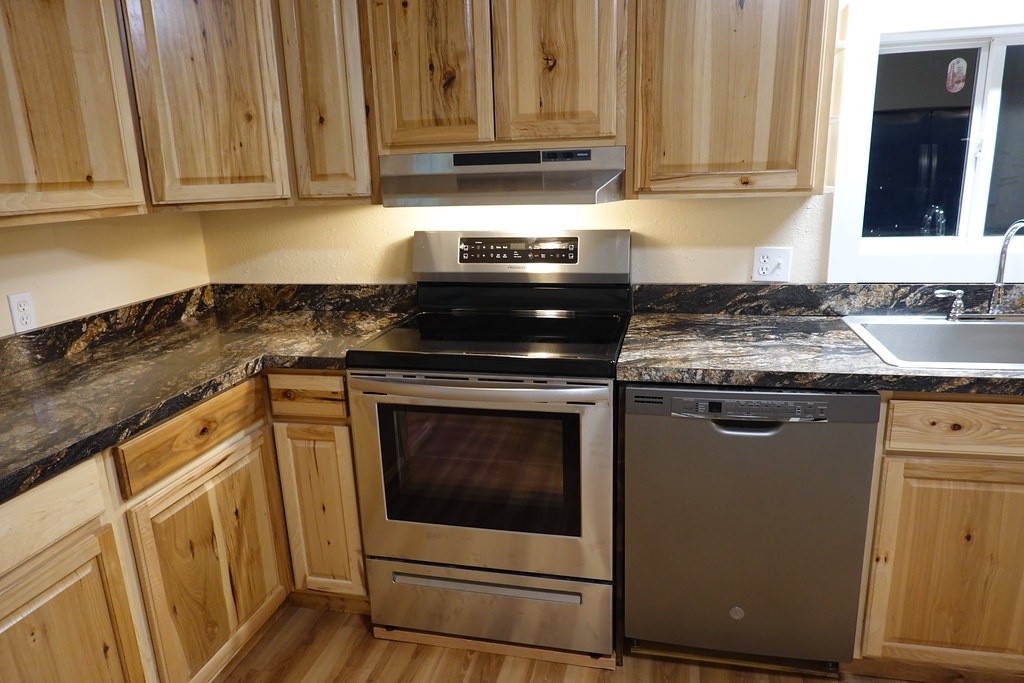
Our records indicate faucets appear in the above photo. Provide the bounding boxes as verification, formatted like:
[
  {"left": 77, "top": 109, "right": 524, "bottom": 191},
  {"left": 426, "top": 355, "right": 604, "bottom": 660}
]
[
  {"left": 920, "top": 205, "right": 945, "bottom": 236},
  {"left": 991, "top": 219, "right": 1024, "bottom": 312}
]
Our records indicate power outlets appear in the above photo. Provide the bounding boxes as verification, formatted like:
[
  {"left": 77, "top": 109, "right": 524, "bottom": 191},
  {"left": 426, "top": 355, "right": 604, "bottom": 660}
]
[
  {"left": 752, "top": 247, "right": 792, "bottom": 283},
  {"left": 8, "top": 291, "right": 35, "bottom": 334}
]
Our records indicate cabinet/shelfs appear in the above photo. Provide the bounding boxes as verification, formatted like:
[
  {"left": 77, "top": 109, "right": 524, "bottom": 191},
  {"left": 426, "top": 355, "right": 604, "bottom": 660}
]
[
  {"left": 0, "top": 369, "right": 369, "bottom": 683},
  {"left": 0, "top": 0, "right": 847, "bottom": 227},
  {"left": 851, "top": 390, "right": 1024, "bottom": 683}
]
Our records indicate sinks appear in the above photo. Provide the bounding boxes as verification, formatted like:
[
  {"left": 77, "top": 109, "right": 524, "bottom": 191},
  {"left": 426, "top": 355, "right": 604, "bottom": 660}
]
[{"left": 847, "top": 313, "right": 1024, "bottom": 367}]
